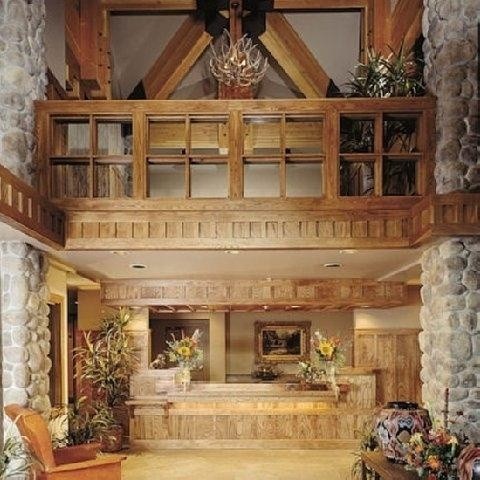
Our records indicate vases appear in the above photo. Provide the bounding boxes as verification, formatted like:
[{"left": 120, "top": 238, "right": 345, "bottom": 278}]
[
  {"left": 324, "top": 358, "right": 334, "bottom": 389},
  {"left": 375, "top": 402, "right": 431, "bottom": 464},
  {"left": 179, "top": 362, "right": 191, "bottom": 393}
]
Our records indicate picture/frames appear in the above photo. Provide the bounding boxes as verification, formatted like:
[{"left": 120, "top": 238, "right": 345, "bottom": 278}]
[{"left": 254, "top": 320, "right": 311, "bottom": 363}]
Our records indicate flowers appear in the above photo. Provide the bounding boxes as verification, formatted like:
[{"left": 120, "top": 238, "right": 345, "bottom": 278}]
[
  {"left": 166, "top": 328, "right": 203, "bottom": 368},
  {"left": 404, "top": 429, "right": 479, "bottom": 480},
  {"left": 310, "top": 329, "right": 345, "bottom": 362},
  {"left": 296, "top": 361, "right": 324, "bottom": 383}
]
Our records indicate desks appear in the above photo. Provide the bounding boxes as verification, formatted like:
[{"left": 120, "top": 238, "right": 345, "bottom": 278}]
[{"left": 362, "top": 450, "right": 420, "bottom": 480}]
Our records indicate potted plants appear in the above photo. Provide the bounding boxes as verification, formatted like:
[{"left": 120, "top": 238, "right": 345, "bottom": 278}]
[{"left": 72, "top": 307, "right": 140, "bottom": 451}]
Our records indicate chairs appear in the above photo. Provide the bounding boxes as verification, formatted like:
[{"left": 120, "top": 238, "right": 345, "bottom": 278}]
[{"left": 3, "top": 405, "right": 126, "bottom": 480}]
